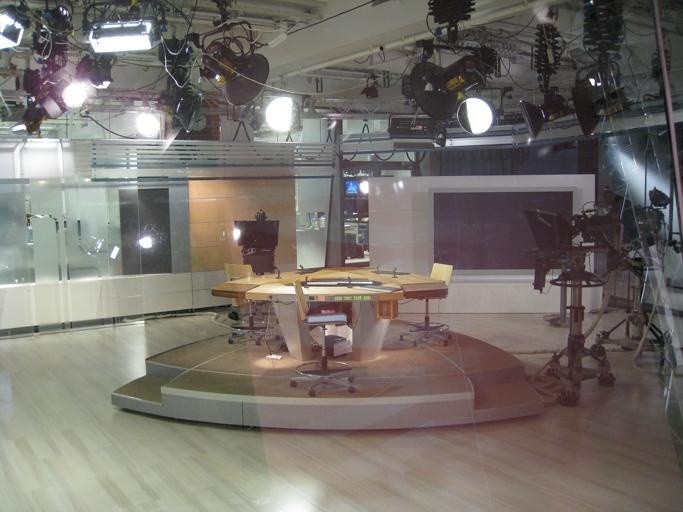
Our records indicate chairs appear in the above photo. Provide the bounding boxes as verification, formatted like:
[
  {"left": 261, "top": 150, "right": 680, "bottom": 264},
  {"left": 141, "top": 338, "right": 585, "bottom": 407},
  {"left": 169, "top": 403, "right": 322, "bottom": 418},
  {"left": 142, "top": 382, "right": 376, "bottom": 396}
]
[
  {"left": 400, "top": 263, "right": 454, "bottom": 348},
  {"left": 224, "top": 264, "right": 271, "bottom": 345},
  {"left": 294, "top": 279, "right": 354, "bottom": 397}
]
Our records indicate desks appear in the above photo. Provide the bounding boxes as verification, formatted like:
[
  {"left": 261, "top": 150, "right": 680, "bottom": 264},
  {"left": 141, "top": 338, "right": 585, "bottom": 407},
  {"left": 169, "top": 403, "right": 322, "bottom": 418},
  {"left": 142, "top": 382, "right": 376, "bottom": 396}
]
[{"left": 211, "top": 267, "right": 448, "bottom": 361}]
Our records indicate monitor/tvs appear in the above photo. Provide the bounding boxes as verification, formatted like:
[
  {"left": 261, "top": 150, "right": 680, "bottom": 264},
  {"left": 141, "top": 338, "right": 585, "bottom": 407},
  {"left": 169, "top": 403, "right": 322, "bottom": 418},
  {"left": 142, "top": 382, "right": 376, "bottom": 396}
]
[
  {"left": 344, "top": 177, "right": 362, "bottom": 197},
  {"left": 426, "top": 186, "right": 578, "bottom": 278}
]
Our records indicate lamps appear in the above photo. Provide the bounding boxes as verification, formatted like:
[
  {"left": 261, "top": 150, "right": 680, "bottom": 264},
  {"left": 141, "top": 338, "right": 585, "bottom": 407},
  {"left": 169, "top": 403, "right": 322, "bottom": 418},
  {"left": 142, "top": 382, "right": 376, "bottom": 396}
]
[
  {"left": 158, "top": 66, "right": 202, "bottom": 133},
  {"left": 520, "top": 88, "right": 575, "bottom": 138},
  {"left": 524, "top": 207, "right": 560, "bottom": 252},
  {"left": 411, "top": 46, "right": 500, "bottom": 120},
  {"left": 16, "top": 0, "right": 89, "bottom": 133},
  {"left": 0, "top": 1, "right": 27, "bottom": 49},
  {"left": 571, "top": 52, "right": 630, "bottom": 137},
  {"left": 201, "top": 21, "right": 270, "bottom": 107},
  {"left": 388, "top": 112, "right": 448, "bottom": 152},
  {"left": 82, "top": 0, "right": 166, "bottom": 53},
  {"left": 87, "top": 54, "right": 114, "bottom": 89}
]
